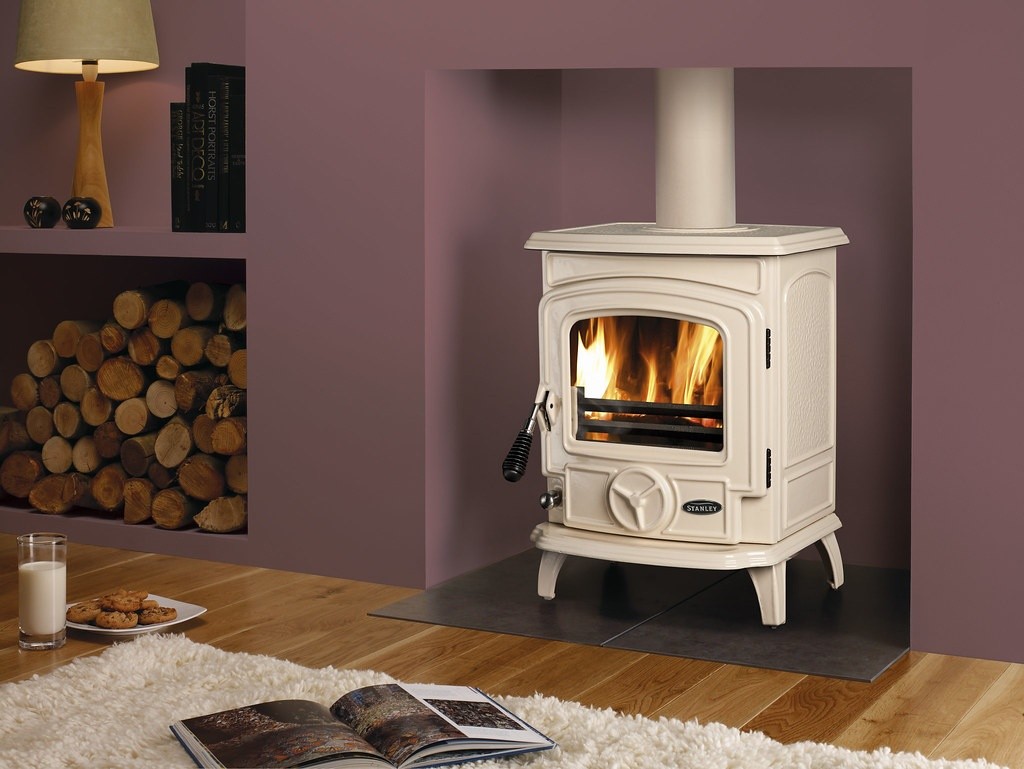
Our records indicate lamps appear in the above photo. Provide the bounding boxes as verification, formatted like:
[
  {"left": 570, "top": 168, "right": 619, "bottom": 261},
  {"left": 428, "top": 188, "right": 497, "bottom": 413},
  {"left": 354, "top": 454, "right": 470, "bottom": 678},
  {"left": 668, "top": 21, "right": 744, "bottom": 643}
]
[{"left": 13, "top": 0, "right": 159, "bottom": 228}]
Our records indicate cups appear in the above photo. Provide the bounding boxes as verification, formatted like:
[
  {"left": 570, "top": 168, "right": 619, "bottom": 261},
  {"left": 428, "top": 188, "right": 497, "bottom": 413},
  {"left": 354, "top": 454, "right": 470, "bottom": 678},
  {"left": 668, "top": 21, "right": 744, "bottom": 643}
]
[{"left": 16, "top": 533, "right": 67, "bottom": 651}]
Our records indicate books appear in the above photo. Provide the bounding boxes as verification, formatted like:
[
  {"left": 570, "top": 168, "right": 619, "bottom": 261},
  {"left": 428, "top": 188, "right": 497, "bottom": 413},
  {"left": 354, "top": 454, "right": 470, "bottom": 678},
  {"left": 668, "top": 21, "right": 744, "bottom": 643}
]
[
  {"left": 169, "top": 61, "right": 247, "bottom": 233},
  {"left": 169, "top": 683, "right": 559, "bottom": 768}
]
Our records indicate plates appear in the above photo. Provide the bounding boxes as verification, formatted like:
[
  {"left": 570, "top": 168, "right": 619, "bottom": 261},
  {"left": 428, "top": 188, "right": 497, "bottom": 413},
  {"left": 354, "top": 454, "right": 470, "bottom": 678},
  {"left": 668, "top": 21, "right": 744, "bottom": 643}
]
[{"left": 66, "top": 594, "right": 207, "bottom": 636}]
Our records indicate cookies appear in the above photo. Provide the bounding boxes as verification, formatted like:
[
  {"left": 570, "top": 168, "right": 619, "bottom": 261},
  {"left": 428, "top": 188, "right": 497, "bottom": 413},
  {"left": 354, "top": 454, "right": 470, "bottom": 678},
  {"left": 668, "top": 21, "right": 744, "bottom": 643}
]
[{"left": 66, "top": 589, "right": 176, "bottom": 629}]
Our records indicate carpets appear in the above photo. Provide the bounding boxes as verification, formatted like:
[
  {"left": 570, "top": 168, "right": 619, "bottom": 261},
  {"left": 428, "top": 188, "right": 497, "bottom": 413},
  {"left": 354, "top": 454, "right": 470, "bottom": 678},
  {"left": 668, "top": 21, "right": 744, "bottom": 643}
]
[
  {"left": 367, "top": 558, "right": 911, "bottom": 681},
  {"left": 0, "top": 633, "right": 1012, "bottom": 769}
]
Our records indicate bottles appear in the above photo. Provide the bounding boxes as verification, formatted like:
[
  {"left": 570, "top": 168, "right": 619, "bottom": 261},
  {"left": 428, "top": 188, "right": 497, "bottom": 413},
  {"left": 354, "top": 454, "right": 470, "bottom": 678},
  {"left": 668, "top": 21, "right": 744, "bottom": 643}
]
[
  {"left": 23, "top": 196, "right": 62, "bottom": 228},
  {"left": 61, "top": 197, "right": 102, "bottom": 229}
]
